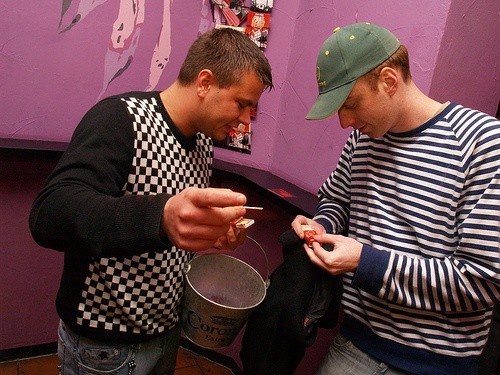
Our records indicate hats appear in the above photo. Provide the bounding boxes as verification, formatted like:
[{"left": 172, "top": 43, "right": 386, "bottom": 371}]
[{"left": 305, "top": 22, "right": 401, "bottom": 121}]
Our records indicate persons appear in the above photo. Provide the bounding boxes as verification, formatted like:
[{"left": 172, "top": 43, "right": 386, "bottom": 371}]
[
  {"left": 290, "top": 22, "right": 500, "bottom": 375},
  {"left": 29, "top": 28, "right": 276, "bottom": 374}
]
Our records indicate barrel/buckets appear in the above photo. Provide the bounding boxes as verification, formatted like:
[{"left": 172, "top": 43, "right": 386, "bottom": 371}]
[{"left": 183, "top": 233, "right": 272, "bottom": 350}]
